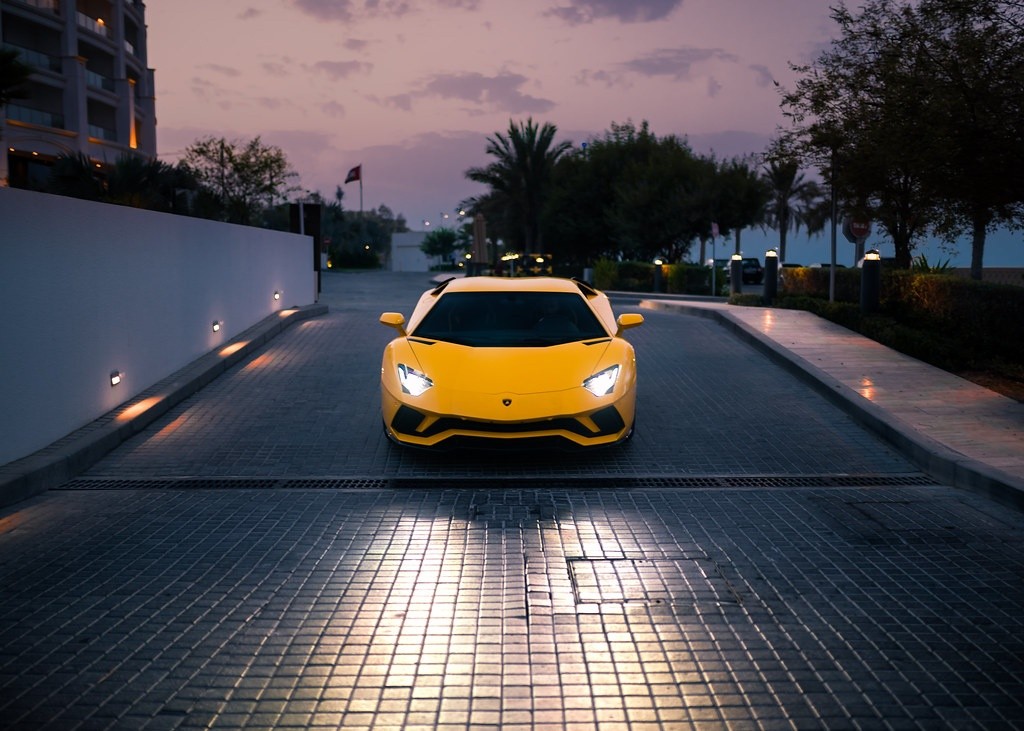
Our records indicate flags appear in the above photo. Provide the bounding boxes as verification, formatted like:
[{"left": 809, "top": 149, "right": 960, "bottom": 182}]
[{"left": 345, "top": 167, "right": 360, "bottom": 184}]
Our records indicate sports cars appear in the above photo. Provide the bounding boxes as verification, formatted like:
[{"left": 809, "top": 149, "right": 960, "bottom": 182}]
[{"left": 378, "top": 275, "right": 645, "bottom": 454}]
